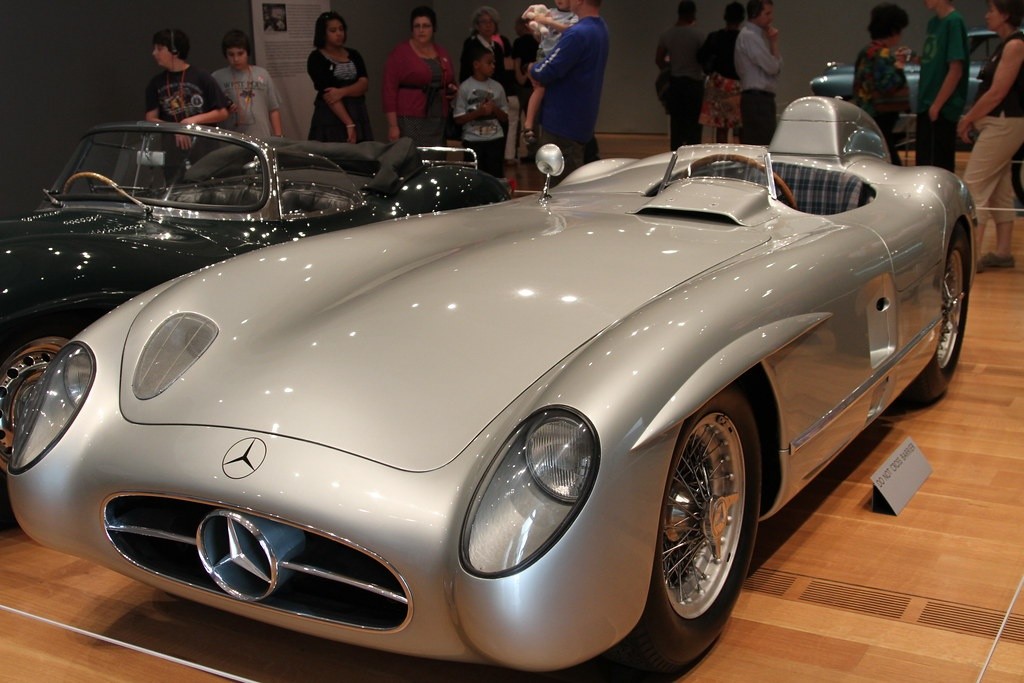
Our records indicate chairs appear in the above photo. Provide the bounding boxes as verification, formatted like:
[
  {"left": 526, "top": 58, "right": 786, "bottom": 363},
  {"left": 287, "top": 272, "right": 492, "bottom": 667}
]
[{"left": 675, "top": 162, "right": 861, "bottom": 221}]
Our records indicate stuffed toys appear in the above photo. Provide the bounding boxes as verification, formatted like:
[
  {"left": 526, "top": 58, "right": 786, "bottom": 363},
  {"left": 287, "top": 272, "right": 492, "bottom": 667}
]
[{"left": 522, "top": 4, "right": 551, "bottom": 35}]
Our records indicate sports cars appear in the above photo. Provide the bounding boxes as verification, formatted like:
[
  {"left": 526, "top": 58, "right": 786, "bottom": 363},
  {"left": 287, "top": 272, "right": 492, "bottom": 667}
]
[
  {"left": 0, "top": 122, "right": 523, "bottom": 533},
  {"left": 9, "top": 96, "right": 980, "bottom": 683}
]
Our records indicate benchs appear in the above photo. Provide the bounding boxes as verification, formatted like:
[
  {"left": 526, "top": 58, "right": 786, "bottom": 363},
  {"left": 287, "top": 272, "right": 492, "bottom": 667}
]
[{"left": 187, "top": 133, "right": 422, "bottom": 200}]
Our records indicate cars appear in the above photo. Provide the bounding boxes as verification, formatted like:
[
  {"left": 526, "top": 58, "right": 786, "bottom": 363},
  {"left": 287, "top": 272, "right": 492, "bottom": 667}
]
[{"left": 806, "top": 29, "right": 1024, "bottom": 143}]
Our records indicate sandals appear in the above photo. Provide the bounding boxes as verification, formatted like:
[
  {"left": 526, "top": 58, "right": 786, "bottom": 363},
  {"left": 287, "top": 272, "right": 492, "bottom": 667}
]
[{"left": 523, "top": 124, "right": 537, "bottom": 144}]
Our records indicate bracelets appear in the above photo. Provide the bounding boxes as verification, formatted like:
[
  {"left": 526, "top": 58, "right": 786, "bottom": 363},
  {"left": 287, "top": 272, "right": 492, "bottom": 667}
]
[
  {"left": 346, "top": 123, "right": 356, "bottom": 129},
  {"left": 275, "top": 134, "right": 285, "bottom": 138}
]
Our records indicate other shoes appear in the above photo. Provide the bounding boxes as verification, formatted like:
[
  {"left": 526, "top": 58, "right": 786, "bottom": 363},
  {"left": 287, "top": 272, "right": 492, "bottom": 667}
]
[{"left": 981, "top": 251, "right": 1015, "bottom": 267}]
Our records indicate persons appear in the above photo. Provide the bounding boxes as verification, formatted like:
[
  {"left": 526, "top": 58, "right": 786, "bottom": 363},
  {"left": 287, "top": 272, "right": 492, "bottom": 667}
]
[
  {"left": 521, "top": 0, "right": 609, "bottom": 189},
  {"left": 144, "top": 28, "right": 229, "bottom": 186},
  {"left": 211, "top": 29, "right": 286, "bottom": 148},
  {"left": 735, "top": 0, "right": 783, "bottom": 145},
  {"left": 381, "top": 6, "right": 458, "bottom": 160},
  {"left": 453, "top": 8, "right": 540, "bottom": 177},
  {"left": 915, "top": 0, "right": 970, "bottom": 173},
  {"left": 698, "top": 2, "right": 746, "bottom": 144},
  {"left": 958, "top": 0, "right": 1024, "bottom": 272},
  {"left": 655, "top": 0, "right": 706, "bottom": 153},
  {"left": 307, "top": 10, "right": 374, "bottom": 143},
  {"left": 852, "top": 3, "right": 911, "bottom": 166}
]
